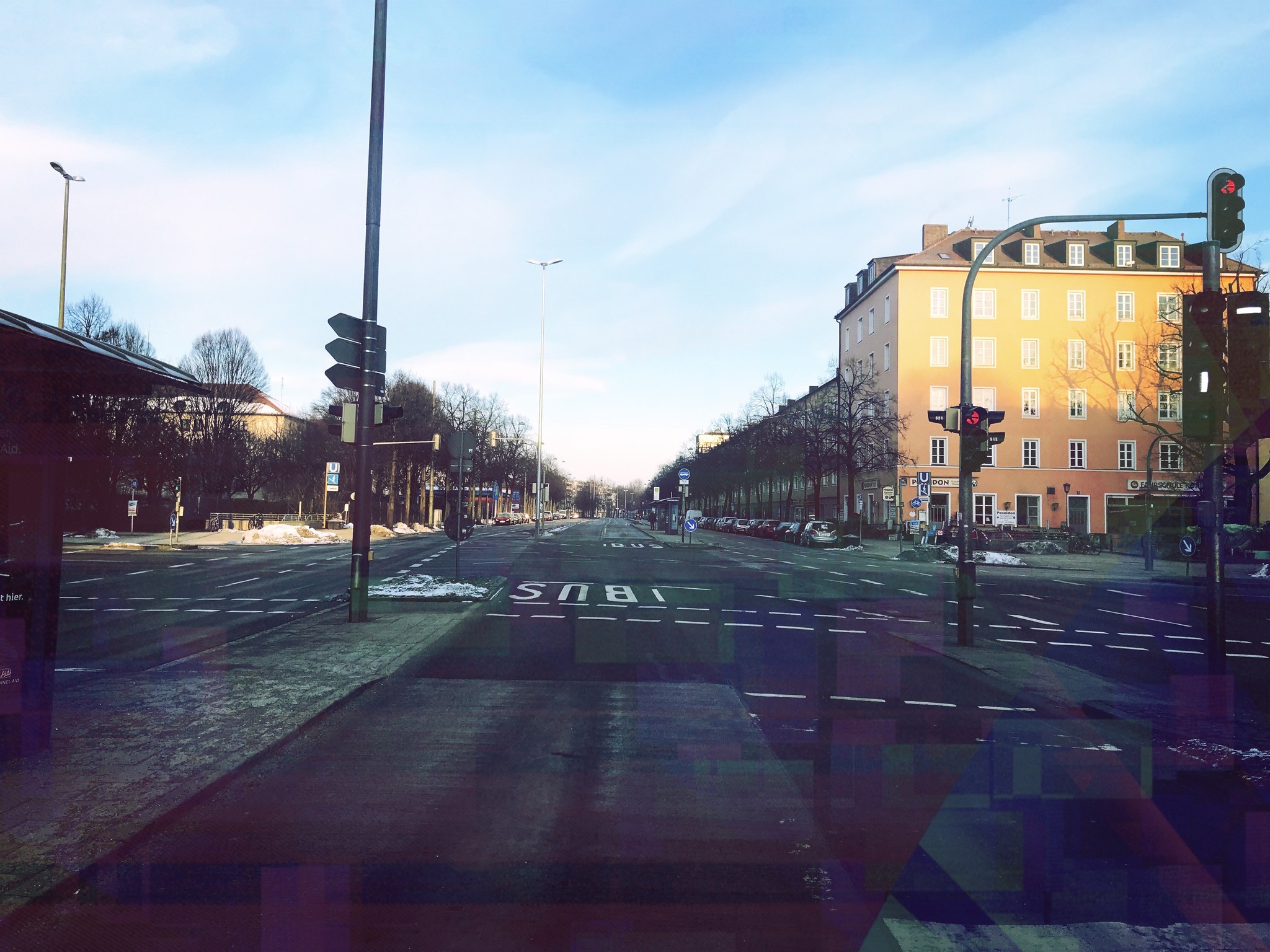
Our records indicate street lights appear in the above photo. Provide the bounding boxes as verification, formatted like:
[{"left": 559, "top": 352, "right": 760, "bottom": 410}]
[
  {"left": 524, "top": 257, "right": 565, "bottom": 541},
  {"left": 623, "top": 490, "right": 635, "bottom": 511},
  {"left": 1063, "top": 483, "right": 1072, "bottom": 531},
  {"left": 49, "top": 160, "right": 86, "bottom": 330}
]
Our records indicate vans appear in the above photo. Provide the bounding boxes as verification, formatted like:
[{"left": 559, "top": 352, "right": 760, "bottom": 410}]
[
  {"left": 686, "top": 510, "right": 702, "bottom": 522},
  {"left": 558, "top": 510, "right": 567, "bottom": 519},
  {"left": 544, "top": 511, "right": 552, "bottom": 515}
]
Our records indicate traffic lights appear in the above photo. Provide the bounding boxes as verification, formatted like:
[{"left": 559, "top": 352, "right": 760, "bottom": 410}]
[
  {"left": 928, "top": 407, "right": 957, "bottom": 432},
  {"left": 327, "top": 400, "right": 358, "bottom": 446},
  {"left": 529, "top": 483, "right": 536, "bottom": 493},
  {"left": 1180, "top": 290, "right": 1270, "bottom": 441},
  {"left": 374, "top": 401, "right": 404, "bottom": 427},
  {"left": 1207, "top": 167, "right": 1246, "bottom": 253},
  {"left": 540, "top": 483, "right": 548, "bottom": 489},
  {"left": 960, "top": 406, "right": 1007, "bottom": 472}
]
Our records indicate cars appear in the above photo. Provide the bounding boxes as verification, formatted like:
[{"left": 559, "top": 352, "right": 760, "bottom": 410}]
[
  {"left": 634, "top": 514, "right": 642, "bottom": 521},
  {"left": 570, "top": 513, "right": 579, "bottom": 519},
  {"left": 693, "top": 516, "right": 839, "bottom": 548},
  {"left": 532, "top": 513, "right": 554, "bottom": 522},
  {"left": 643, "top": 515, "right": 648, "bottom": 520},
  {"left": 495, "top": 512, "right": 530, "bottom": 525},
  {"left": 666, "top": 514, "right": 684, "bottom": 524},
  {"left": 552, "top": 513, "right": 560, "bottom": 520}
]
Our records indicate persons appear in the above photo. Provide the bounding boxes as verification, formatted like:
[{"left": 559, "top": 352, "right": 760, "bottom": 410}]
[{"left": 649, "top": 511, "right": 655, "bottom": 531}]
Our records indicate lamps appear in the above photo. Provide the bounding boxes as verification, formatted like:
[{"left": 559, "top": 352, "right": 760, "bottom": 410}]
[
  {"left": 872, "top": 500, "right": 879, "bottom": 508},
  {"left": 1052, "top": 502, "right": 1059, "bottom": 512},
  {"left": 832, "top": 502, "right": 842, "bottom": 509},
  {"left": 1004, "top": 501, "right": 1012, "bottom": 511}
]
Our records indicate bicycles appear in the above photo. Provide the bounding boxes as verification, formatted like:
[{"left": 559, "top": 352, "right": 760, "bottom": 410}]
[{"left": 1066, "top": 533, "right": 1103, "bottom": 555}]
